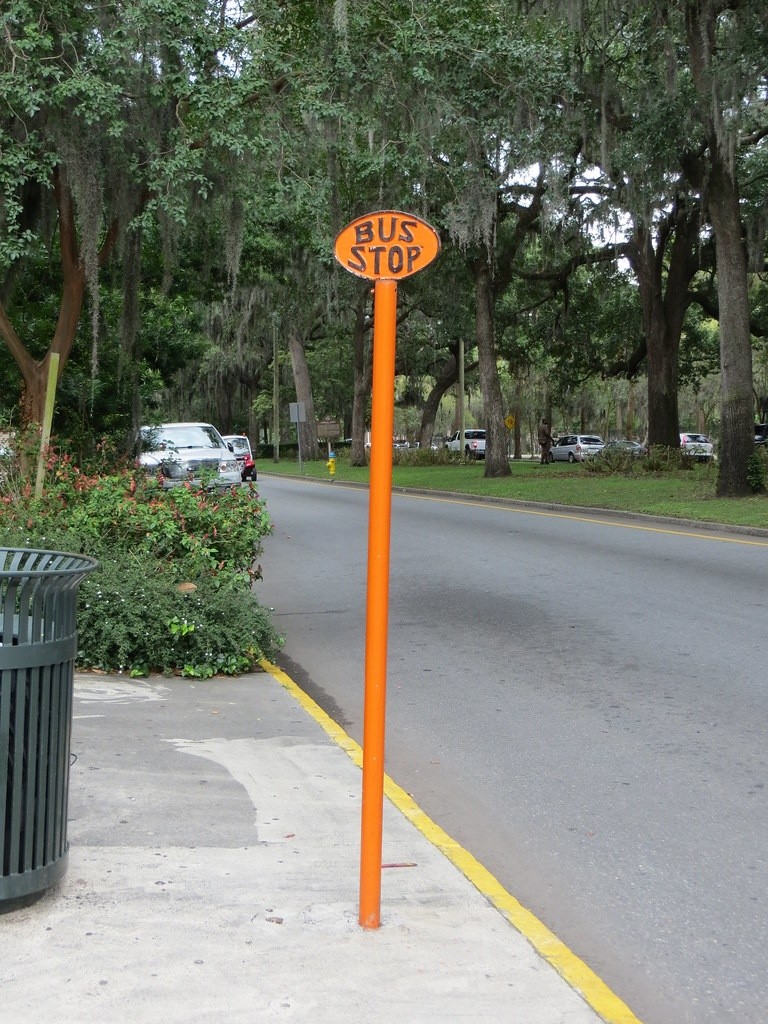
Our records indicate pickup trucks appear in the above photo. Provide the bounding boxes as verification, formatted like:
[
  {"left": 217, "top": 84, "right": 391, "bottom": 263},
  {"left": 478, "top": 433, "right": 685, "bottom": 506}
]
[{"left": 444, "top": 429, "right": 487, "bottom": 460}]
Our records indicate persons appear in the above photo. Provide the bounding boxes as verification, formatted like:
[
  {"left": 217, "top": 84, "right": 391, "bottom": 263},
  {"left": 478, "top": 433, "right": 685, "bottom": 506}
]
[{"left": 538, "top": 417, "right": 556, "bottom": 464}]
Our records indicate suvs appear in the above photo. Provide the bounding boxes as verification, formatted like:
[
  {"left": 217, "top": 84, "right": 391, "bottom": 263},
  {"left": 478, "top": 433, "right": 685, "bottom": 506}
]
[{"left": 133, "top": 422, "right": 242, "bottom": 502}]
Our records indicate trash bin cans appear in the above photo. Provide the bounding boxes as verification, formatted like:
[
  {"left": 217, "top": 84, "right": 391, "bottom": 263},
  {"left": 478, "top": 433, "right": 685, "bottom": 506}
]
[{"left": 0, "top": 546, "right": 101, "bottom": 902}]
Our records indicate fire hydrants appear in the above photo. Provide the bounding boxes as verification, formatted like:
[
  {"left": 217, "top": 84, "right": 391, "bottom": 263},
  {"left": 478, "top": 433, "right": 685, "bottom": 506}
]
[{"left": 326, "top": 452, "right": 337, "bottom": 478}]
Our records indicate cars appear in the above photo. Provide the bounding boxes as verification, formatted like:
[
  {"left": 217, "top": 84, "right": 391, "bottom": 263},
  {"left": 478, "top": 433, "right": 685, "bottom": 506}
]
[
  {"left": 220, "top": 434, "right": 257, "bottom": 482},
  {"left": 548, "top": 435, "right": 606, "bottom": 463},
  {"left": 593, "top": 441, "right": 648, "bottom": 466},
  {"left": 755, "top": 423, "right": 768, "bottom": 446},
  {"left": 680, "top": 433, "right": 713, "bottom": 463},
  {"left": 344, "top": 438, "right": 439, "bottom": 456}
]
